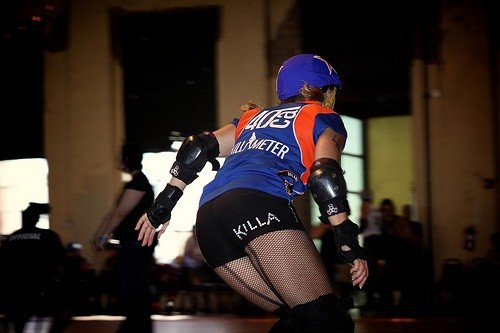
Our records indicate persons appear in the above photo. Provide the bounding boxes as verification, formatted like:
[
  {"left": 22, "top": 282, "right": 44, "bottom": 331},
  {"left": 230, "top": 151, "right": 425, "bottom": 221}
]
[
  {"left": 302, "top": 192, "right": 442, "bottom": 318},
  {"left": 133, "top": 53, "right": 371, "bottom": 332},
  {"left": 1, "top": 205, "right": 211, "bottom": 332},
  {"left": 91, "top": 141, "right": 156, "bottom": 333}
]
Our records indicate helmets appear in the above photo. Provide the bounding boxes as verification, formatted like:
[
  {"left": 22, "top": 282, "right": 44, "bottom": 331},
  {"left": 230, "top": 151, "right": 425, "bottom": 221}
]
[{"left": 275, "top": 54, "right": 341, "bottom": 100}]
